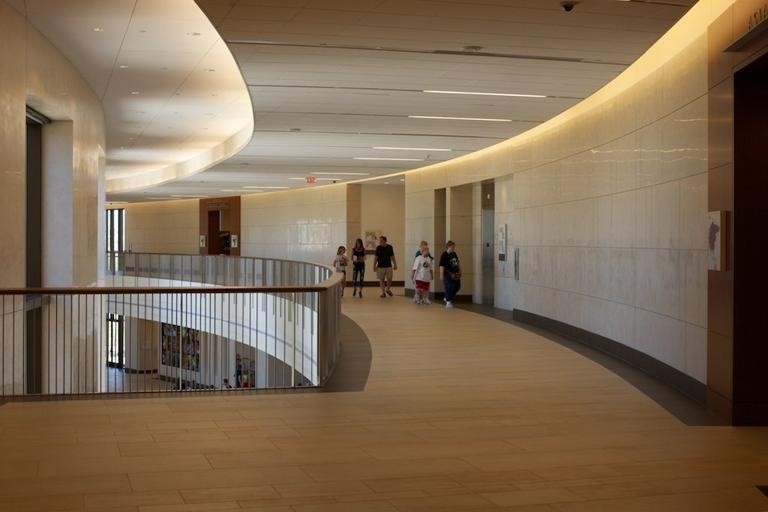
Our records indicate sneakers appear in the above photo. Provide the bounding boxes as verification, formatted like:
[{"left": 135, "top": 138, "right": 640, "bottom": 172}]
[
  {"left": 353, "top": 289, "right": 394, "bottom": 299},
  {"left": 446, "top": 301, "right": 454, "bottom": 309}
]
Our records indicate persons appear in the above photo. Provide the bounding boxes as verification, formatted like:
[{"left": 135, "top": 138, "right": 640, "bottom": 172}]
[
  {"left": 439, "top": 240, "right": 461, "bottom": 309},
  {"left": 236, "top": 364, "right": 242, "bottom": 387},
  {"left": 351, "top": 238, "right": 367, "bottom": 298},
  {"left": 173, "top": 375, "right": 231, "bottom": 389},
  {"left": 412, "top": 241, "right": 432, "bottom": 301},
  {"left": 333, "top": 246, "right": 349, "bottom": 296},
  {"left": 410, "top": 247, "right": 434, "bottom": 305},
  {"left": 373, "top": 236, "right": 398, "bottom": 298}
]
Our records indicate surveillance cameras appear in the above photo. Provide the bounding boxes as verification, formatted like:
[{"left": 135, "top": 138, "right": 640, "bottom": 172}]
[{"left": 558, "top": 1, "right": 579, "bottom": 13}]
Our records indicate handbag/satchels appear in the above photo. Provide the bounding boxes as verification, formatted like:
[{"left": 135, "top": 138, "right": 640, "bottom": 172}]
[{"left": 452, "top": 272, "right": 461, "bottom": 280}]
